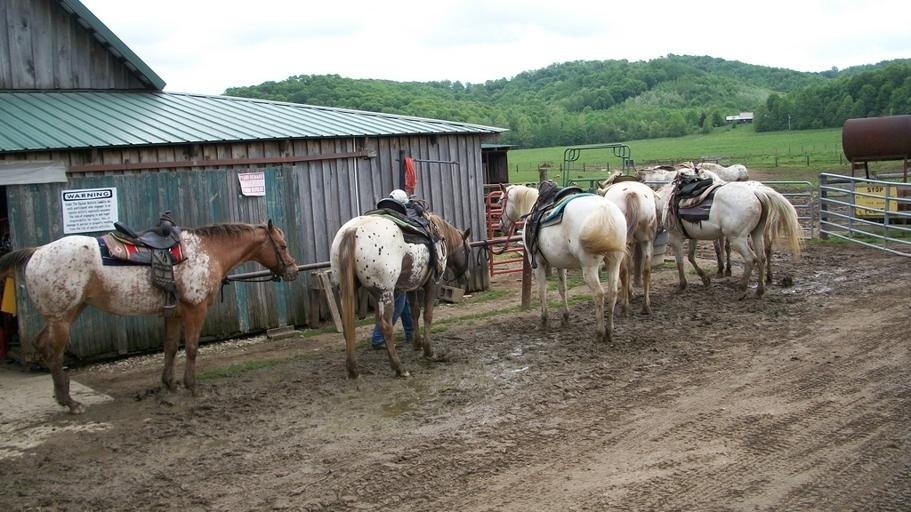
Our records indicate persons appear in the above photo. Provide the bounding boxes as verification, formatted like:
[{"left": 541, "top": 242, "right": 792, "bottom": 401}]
[{"left": 369, "top": 187, "right": 426, "bottom": 351}]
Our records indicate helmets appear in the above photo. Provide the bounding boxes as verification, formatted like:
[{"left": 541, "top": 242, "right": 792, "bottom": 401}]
[{"left": 388, "top": 190, "right": 411, "bottom": 206}]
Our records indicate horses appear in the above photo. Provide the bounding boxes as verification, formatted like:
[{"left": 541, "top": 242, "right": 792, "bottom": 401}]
[
  {"left": 595, "top": 161, "right": 806, "bottom": 318},
  {"left": 0, "top": 217, "right": 301, "bottom": 415},
  {"left": 497, "top": 181, "right": 631, "bottom": 344},
  {"left": 329, "top": 203, "right": 472, "bottom": 381}
]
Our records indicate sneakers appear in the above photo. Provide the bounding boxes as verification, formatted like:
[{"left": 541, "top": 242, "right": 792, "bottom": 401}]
[{"left": 373, "top": 342, "right": 397, "bottom": 351}]
